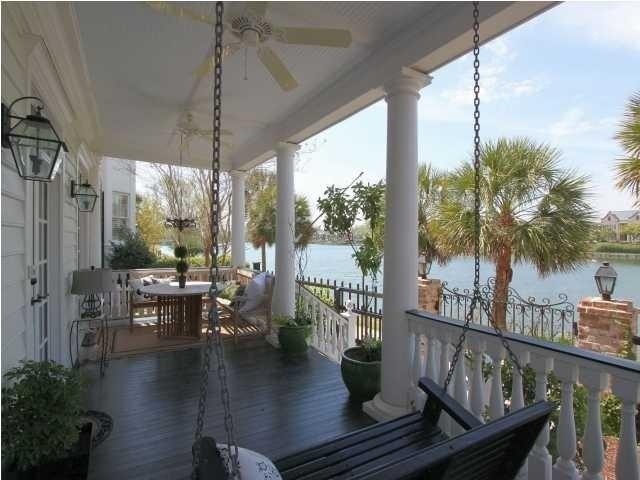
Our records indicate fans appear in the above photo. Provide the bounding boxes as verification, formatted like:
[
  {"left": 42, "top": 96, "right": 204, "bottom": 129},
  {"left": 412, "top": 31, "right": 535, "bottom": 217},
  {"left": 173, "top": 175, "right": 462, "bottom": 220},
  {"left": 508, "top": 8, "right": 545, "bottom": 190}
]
[
  {"left": 167, "top": 115, "right": 234, "bottom": 151},
  {"left": 143, "top": 1, "right": 351, "bottom": 92}
]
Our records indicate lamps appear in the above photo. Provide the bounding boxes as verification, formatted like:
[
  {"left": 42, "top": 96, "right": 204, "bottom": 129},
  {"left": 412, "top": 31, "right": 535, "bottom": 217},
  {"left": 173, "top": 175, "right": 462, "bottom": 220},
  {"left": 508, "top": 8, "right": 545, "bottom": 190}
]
[
  {"left": 72, "top": 177, "right": 99, "bottom": 213},
  {"left": 1, "top": 96, "right": 68, "bottom": 183},
  {"left": 70, "top": 266, "right": 112, "bottom": 318}
]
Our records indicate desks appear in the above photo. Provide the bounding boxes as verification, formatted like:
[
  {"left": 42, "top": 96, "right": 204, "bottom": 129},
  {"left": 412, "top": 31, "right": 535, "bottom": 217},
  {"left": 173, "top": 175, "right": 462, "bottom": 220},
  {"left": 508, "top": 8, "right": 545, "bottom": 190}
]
[
  {"left": 68, "top": 313, "right": 109, "bottom": 378},
  {"left": 140, "top": 281, "right": 225, "bottom": 340}
]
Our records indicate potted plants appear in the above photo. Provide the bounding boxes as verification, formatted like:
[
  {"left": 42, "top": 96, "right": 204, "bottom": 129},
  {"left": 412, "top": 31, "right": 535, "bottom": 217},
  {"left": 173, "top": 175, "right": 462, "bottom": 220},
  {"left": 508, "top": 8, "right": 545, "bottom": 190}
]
[
  {"left": 256, "top": 193, "right": 315, "bottom": 354},
  {"left": 1, "top": 357, "right": 93, "bottom": 480},
  {"left": 174, "top": 245, "right": 189, "bottom": 288},
  {"left": 315, "top": 175, "right": 385, "bottom": 405}
]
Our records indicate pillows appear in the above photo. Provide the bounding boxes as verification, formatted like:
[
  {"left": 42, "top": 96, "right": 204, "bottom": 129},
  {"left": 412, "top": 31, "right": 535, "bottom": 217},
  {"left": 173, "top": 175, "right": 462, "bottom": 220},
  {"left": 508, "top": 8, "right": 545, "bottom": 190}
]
[
  {"left": 219, "top": 271, "right": 269, "bottom": 314},
  {"left": 128, "top": 274, "right": 171, "bottom": 303},
  {"left": 214, "top": 442, "right": 282, "bottom": 480}
]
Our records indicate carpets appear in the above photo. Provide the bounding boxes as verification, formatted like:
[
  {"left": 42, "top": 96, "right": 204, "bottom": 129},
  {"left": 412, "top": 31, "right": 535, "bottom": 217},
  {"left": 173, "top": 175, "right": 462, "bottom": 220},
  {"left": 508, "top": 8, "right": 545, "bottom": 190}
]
[
  {"left": 78, "top": 312, "right": 265, "bottom": 363},
  {"left": 76, "top": 409, "right": 114, "bottom": 451}
]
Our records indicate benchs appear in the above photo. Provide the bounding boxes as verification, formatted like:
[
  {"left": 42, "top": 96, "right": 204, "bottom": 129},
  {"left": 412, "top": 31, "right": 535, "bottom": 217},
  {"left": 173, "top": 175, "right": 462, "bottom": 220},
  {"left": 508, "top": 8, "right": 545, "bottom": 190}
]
[
  {"left": 127, "top": 266, "right": 239, "bottom": 332},
  {"left": 216, "top": 269, "right": 276, "bottom": 344}
]
